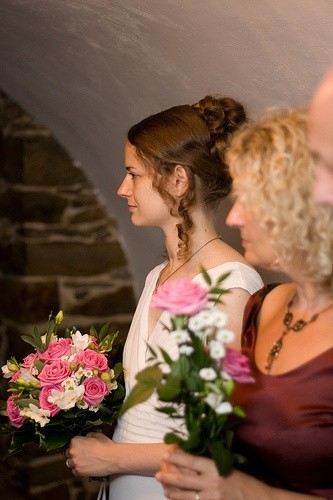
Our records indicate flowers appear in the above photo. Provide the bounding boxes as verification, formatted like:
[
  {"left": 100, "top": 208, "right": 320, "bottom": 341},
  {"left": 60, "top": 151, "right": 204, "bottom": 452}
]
[
  {"left": 0, "top": 310, "right": 126, "bottom": 481},
  {"left": 119, "top": 265, "right": 255, "bottom": 478}
]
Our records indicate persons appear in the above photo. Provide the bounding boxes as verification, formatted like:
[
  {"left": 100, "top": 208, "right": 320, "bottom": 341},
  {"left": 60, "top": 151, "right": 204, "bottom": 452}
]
[
  {"left": 66, "top": 95, "right": 267, "bottom": 500},
  {"left": 155, "top": 104, "right": 333, "bottom": 500}
]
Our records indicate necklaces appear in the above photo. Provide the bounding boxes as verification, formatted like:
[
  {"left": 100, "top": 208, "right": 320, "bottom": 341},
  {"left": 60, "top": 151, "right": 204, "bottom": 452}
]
[
  {"left": 265, "top": 292, "right": 333, "bottom": 374},
  {"left": 153, "top": 236, "right": 223, "bottom": 293}
]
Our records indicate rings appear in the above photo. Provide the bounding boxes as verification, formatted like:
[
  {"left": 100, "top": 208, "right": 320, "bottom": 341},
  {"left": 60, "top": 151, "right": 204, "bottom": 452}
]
[
  {"left": 66, "top": 459, "right": 71, "bottom": 469},
  {"left": 196, "top": 491, "right": 200, "bottom": 500}
]
